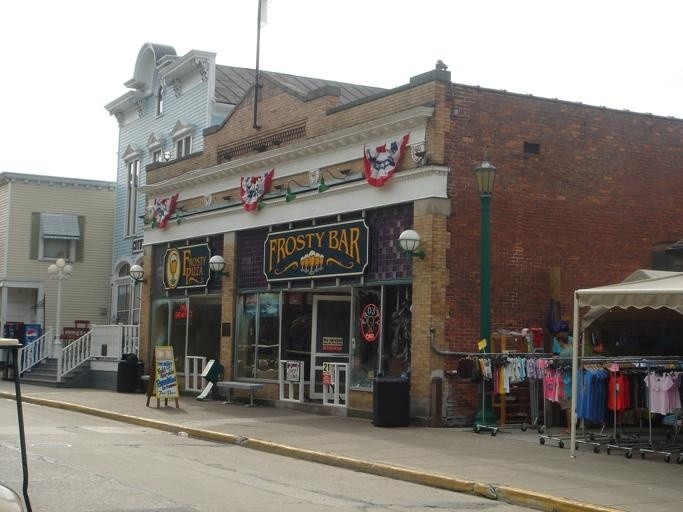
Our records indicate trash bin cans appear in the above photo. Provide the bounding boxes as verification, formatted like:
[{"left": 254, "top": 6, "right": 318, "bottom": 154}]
[
  {"left": 118, "top": 360, "right": 138, "bottom": 393},
  {"left": 374, "top": 376, "right": 410, "bottom": 427}
]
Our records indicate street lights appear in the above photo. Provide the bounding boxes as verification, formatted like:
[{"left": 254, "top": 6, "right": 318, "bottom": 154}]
[
  {"left": 474, "top": 159, "right": 497, "bottom": 426},
  {"left": 48, "top": 258, "right": 72, "bottom": 358}
]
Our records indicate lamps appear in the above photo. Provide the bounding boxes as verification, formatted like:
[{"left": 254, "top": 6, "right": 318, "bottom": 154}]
[
  {"left": 209, "top": 255, "right": 229, "bottom": 277},
  {"left": 129, "top": 264, "right": 148, "bottom": 284},
  {"left": 397, "top": 230, "right": 426, "bottom": 259}
]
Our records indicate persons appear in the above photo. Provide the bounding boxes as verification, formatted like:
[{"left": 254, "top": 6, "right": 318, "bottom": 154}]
[{"left": 556, "top": 331, "right": 572, "bottom": 435}]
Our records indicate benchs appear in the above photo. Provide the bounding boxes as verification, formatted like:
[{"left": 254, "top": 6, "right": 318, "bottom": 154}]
[{"left": 216, "top": 381, "right": 263, "bottom": 408}]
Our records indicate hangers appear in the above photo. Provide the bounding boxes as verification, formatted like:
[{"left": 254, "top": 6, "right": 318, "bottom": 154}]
[
  {"left": 458, "top": 350, "right": 550, "bottom": 365},
  {"left": 542, "top": 351, "right": 680, "bottom": 379}
]
